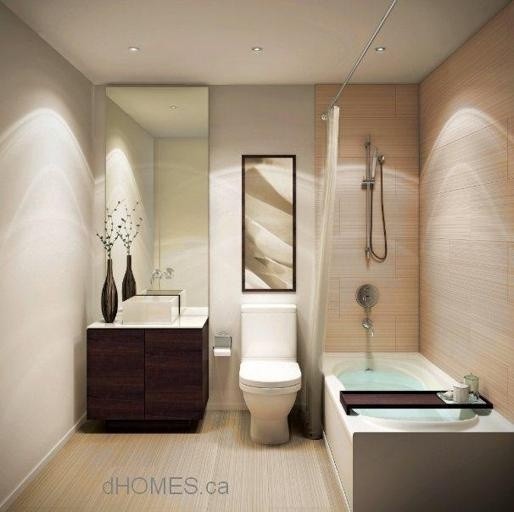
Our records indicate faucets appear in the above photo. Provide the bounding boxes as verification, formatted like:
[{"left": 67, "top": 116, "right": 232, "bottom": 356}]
[
  {"left": 151, "top": 269, "right": 160, "bottom": 282},
  {"left": 162, "top": 266, "right": 174, "bottom": 281},
  {"left": 362, "top": 321, "right": 375, "bottom": 337}
]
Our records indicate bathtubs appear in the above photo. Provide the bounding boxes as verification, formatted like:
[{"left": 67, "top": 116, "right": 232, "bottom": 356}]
[{"left": 322, "top": 352, "right": 513, "bottom": 512}]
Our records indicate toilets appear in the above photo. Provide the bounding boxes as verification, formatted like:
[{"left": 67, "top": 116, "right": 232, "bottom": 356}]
[{"left": 239, "top": 303, "right": 302, "bottom": 445}]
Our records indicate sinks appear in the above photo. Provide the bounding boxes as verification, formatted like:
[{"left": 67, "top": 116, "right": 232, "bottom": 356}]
[
  {"left": 138, "top": 289, "right": 185, "bottom": 315},
  {"left": 118, "top": 294, "right": 179, "bottom": 323}
]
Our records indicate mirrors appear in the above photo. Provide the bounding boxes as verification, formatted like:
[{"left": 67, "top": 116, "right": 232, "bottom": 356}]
[
  {"left": 242, "top": 154, "right": 296, "bottom": 293},
  {"left": 104, "top": 84, "right": 209, "bottom": 317}
]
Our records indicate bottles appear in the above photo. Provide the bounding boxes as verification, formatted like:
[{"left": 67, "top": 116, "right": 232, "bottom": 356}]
[{"left": 463, "top": 372, "right": 480, "bottom": 402}]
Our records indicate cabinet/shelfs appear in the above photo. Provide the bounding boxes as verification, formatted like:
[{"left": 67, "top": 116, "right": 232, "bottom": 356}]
[{"left": 87, "top": 320, "right": 209, "bottom": 432}]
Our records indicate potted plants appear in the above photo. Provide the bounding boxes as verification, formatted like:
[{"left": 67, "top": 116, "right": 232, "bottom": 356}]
[
  {"left": 118, "top": 200, "right": 143, "bottom": 301},
  {"left": 97, "top": 199, "right": 121, "bottom": 323}
]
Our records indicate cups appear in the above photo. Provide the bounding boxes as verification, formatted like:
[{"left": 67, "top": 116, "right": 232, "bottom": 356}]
[{"left": 452, "top": 384, "right": 470, "bottom": 404}]
